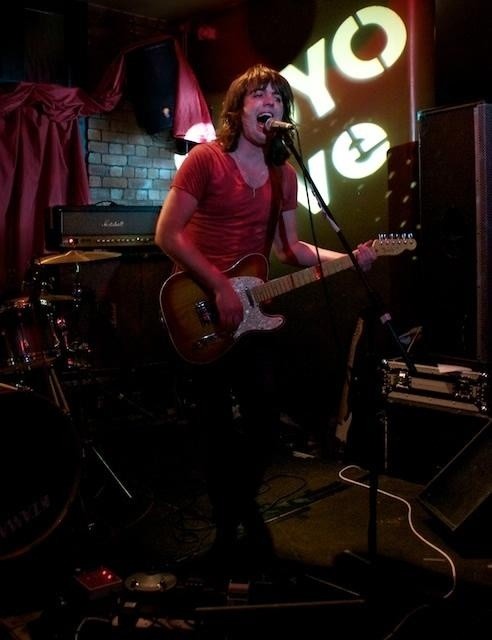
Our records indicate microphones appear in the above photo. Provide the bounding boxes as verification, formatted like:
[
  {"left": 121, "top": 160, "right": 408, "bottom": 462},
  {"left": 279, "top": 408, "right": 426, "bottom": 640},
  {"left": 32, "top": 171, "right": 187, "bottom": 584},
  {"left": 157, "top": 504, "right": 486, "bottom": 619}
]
[{"left": 264, "top": 118, "right": 294, "bottom": 133}]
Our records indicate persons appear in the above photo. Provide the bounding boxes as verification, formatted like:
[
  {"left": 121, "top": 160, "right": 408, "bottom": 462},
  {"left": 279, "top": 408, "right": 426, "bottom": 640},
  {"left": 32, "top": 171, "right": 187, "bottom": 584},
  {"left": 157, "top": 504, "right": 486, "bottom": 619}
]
[{"left": 155, "top": 64, "right": 382, "bottom": 544}]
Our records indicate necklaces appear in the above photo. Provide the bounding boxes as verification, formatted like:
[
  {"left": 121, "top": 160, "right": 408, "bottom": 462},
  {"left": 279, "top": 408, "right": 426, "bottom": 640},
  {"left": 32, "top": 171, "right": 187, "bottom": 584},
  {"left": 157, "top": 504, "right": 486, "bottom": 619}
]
[{"left": 233, "top": 149, "right": 269, "bottom": 200}]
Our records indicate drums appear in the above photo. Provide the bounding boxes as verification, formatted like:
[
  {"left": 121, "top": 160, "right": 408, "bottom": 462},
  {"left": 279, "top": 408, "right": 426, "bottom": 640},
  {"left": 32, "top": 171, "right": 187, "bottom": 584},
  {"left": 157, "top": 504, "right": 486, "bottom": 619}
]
[
  {"left": 0, "top": 384, "right": 82, "bottom": 560},
  {"left": 45, "top": 294, "right": 96, "bottom": 373},
  {"left": 0, "top": 297, "right": 61, "bottom": 374}
]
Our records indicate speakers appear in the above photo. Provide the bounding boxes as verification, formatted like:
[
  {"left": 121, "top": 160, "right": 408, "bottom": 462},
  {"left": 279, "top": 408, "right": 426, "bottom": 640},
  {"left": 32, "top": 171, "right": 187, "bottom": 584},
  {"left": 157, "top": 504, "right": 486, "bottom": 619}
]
[
  {"left": 416, "top": 101, "right": 491, "bottom": 361},
  {"left": 415, "top": 421, "right": 491, "bottom": 553}
]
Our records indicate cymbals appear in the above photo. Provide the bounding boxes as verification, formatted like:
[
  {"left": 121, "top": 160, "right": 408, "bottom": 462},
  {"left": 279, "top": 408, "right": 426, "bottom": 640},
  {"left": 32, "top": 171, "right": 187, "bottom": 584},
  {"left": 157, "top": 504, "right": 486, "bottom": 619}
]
[{"left": 34, "top": 249, "right": 122, "bottom": 264}]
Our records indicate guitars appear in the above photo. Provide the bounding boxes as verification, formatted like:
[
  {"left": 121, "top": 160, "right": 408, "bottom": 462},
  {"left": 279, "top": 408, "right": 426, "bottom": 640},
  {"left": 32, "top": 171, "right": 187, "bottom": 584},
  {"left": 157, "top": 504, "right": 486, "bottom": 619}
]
[
  {"left": 320, "top": 318, "right": 364, "bottom": 465},
  {"left": 158, "top": 232, "right": 415, "bottom": 361}
]
